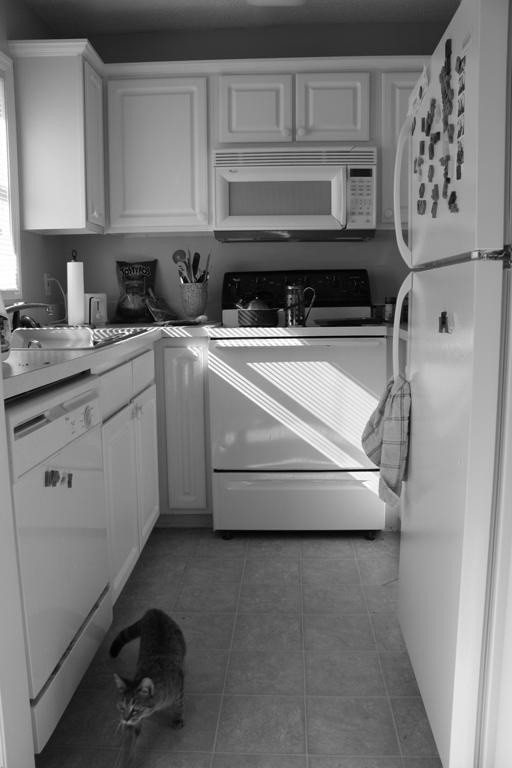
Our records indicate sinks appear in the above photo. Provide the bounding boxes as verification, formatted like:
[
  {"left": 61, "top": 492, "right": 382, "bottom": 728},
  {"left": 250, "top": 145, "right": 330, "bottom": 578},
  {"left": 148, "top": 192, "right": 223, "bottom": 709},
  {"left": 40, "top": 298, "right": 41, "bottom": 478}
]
[{"left": 8, "top": 325, "right": 156, "bottom": 351}]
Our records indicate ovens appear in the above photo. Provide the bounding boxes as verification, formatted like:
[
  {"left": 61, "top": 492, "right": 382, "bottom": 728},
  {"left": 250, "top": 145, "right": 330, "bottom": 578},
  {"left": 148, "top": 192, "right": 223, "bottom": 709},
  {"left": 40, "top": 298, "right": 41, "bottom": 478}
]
[{"left": 12, "top": 421, "right": 109, "bottom": 700}]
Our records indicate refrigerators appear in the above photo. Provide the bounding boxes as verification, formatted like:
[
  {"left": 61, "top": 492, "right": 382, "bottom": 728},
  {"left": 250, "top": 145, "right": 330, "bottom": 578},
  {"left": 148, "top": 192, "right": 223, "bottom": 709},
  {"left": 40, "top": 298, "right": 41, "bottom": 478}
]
[{"left": 392, "top": 0, "right": 512, "bottom": 768}]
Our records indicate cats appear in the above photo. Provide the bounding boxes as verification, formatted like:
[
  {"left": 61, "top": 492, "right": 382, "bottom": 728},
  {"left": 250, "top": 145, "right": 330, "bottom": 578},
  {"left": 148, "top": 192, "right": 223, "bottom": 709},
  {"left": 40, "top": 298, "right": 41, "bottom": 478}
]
[{"left": 108, "top": 607, "right": 186, "bottom": 768}]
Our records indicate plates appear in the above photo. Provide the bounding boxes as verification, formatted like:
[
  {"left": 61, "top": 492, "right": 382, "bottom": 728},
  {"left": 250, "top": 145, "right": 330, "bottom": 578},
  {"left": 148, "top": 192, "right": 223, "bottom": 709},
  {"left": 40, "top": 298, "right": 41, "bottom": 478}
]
[{"left": 313, "top": 316, "right": 373, "bottom": 327}]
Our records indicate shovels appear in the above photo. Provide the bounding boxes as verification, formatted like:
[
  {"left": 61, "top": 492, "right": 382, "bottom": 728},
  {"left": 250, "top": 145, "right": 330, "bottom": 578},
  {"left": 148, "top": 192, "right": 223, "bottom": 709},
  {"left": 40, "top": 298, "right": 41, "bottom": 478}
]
[{"left": 192, "top": 253, "right": 201, "bottom": 276}]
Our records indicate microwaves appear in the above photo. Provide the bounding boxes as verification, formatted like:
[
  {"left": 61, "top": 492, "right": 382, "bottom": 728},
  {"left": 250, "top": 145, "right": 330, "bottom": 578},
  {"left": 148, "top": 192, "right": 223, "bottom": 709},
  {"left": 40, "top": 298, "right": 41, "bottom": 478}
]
[{"left": 211, "top": 144, "right": 379, "bottom": 244}]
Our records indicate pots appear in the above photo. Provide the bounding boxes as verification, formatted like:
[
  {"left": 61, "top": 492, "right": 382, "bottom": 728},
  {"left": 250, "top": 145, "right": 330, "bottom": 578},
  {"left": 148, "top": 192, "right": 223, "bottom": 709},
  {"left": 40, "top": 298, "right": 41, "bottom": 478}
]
[{"left": 236, "top": 295, "right": 280, "bottom": 327}]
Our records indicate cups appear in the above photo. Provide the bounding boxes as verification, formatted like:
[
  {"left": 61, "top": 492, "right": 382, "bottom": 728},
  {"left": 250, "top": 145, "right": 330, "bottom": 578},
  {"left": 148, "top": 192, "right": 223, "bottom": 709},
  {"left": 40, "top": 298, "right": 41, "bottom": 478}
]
[{"left": 285, "top": 284, "right": 316, "bottom": 327}]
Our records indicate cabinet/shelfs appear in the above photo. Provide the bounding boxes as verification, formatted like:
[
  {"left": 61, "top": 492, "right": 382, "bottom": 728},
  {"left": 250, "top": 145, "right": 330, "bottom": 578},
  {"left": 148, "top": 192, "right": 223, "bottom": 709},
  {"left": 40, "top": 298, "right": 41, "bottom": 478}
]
[
  {"left": 157, "top": 331, "right": 211, "bottom": 529},
  {"left": 206, "top": 55, "right": 379, "bottom": 144},
  {"left": 10, "top": 38, "right": 108, "bottom": 234},
  {"left": 92, "top": 332, "right": 160, "bottom": 604},
  {"left": 208, "top": 337, "right": 389, "bottom": 534},
  {"left": 3, "top": 335, "right": 111, "bottom": 758},
  {"left": 105, "top": 41, "right": 214, "bottom": 233},
  {"left": 378, "top": 53, "right": 434, "bottom": 235}
]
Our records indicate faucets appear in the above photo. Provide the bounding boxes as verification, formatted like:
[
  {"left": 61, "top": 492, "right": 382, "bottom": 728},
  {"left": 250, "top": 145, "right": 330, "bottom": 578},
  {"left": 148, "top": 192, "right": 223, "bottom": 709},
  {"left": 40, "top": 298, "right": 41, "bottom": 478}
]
[{"left": 5, "top": 301, "right": 54, "bottom": 315}]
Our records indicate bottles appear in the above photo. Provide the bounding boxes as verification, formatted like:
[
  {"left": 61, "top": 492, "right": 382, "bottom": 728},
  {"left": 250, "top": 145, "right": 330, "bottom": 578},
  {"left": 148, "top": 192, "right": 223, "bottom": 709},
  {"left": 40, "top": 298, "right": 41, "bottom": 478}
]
[{"left": 371, "top": 287, "right": 384, "bottom": 322}]
